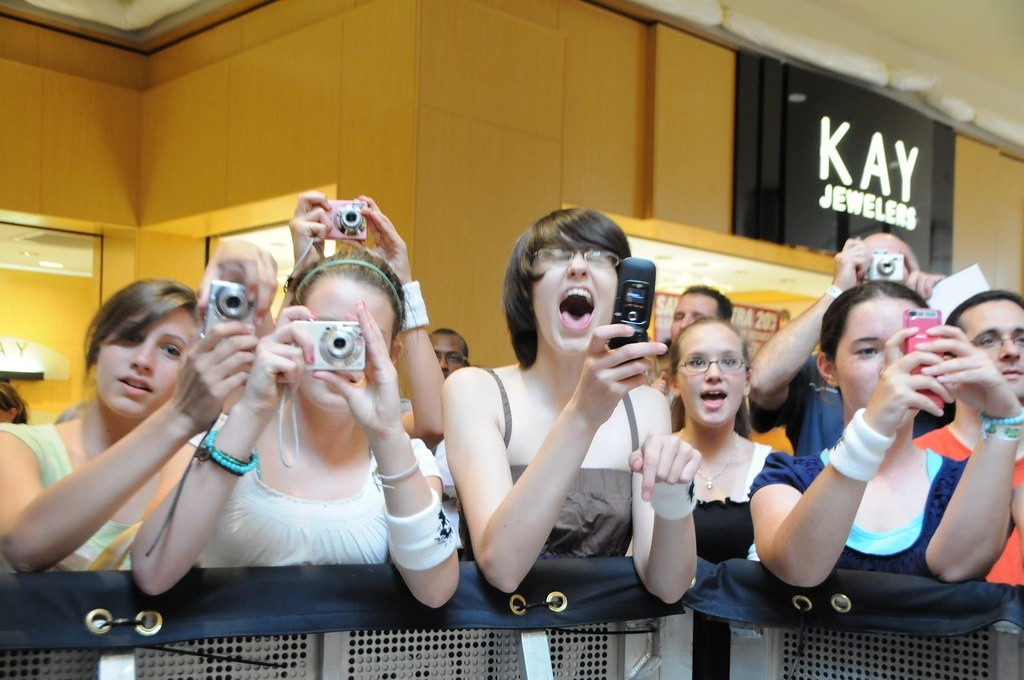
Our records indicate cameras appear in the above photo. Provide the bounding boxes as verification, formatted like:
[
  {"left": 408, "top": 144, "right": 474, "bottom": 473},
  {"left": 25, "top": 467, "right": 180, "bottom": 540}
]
[
  {"left": 291, "top": 319, "right": 367, "bottom": 372},
  {"left": 204, "top": 280, "right": 256, "bottom": 350},
  {"left": 859, "top": 250, "right": 906, "bottom": 281},
  {"left": 313, "top": 199, "right": 369, "bottom": 240}
]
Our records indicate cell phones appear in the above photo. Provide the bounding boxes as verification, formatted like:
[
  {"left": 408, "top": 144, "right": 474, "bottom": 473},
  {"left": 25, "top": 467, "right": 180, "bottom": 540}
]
[
  {"left": 902, "top": 308, "right": 945, "bottom": 408},
  {"left": 608, "top": 258, "right": 656, "bottom": 367}
]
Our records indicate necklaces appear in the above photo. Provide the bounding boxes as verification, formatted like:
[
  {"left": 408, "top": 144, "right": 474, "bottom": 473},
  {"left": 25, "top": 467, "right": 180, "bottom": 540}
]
[{"left": 678, "top": 426, "right": 739, "bottom": 489}]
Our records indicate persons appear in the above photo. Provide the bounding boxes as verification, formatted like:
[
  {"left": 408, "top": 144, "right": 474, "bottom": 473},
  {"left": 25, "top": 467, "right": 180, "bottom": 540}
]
[
  {"left": 747, "top": 279, "right": 1024, "bottom": 587},
  {"left": 278, "top": 190, "right": 472, "bottom": 564},
  {"left": 670, "top": 318, "right": 777, "bottom": 680},
  {"left": 131, "top": 249, "right": 460, "bottom": 608},
  {"left": 0, "top": 240, "right": 276, "bottom": 573},
  {"left": 913, "top": 290, "right": 1024, "bottom": 586},
  {"left": 647, "top": 232, "right": 955, "bottom": 454},
  {"left": 440, "top": 208, "right": 702, "bottom": 604},
  {"left": 0, "top": 380, "right": 93, "bottom": 425}
]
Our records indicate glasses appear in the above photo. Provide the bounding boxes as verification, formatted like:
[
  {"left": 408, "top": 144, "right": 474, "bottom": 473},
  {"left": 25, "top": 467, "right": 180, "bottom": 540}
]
[
  {"left": 971, "top": 333, "right": 1024, "bottom": 348},
  {"left": 681, "top": 357, "right": 746, "bottom": 373},
  {"left": 527, "top": 246, "right": 623, "bottom": 271}
]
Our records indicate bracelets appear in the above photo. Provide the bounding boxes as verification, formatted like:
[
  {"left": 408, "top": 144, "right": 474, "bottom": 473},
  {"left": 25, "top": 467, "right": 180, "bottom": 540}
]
[
  {"left": 375, "top": 457, "right": 419, "bottom": 481},
  {"left": 829, "top": 408, "right": 896, "bottom": 481},
  {"left": 205, "top": 430, "right": 258, "bottom": 476},
  {"left": 650, "top": 482, "right": 697, "bottom": 521},
  {"left": 384, "top": 489, "right": 456, "bottom": 570},
  {"left": 399, "top": 281, "right": 429, "bottom": 332},
  {"left": 979, "top": 408, "right": 1024, "bottom": 441},
  {"left": 826, "top": 283, "right": 843, "bottom": 299}
]
